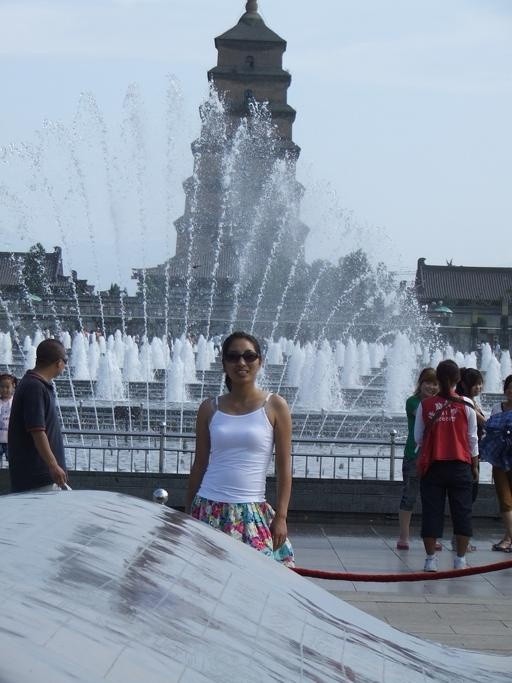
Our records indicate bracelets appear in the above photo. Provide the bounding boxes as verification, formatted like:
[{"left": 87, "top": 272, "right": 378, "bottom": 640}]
[{"left": 273, "top": 512, "right": 287, "bottom": 520}]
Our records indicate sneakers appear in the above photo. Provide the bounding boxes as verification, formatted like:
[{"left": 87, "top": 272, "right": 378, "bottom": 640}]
[
  {"left": 424, "top": 543, "right": 442, "bottom": 571},
  {"left": 453, "top": 542, "right": 472, "bottom": 568},
  {"left": 397, "top": 542, "right": 408, "bottom": 549},
  {"left": 493, "top": 539, "right": 512, "bottom": 552}
]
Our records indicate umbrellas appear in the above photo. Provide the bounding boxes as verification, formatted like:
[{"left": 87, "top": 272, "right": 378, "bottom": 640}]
[{"left": 476, "top": 411, "right": 511, "bottom": 473}]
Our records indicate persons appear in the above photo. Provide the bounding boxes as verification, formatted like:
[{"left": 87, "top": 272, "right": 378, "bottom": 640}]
[
  {"left": 412, "top": 360, "right": 480, "bottom": 572},
  {"left": 7, "top": 337, "right": 70, "bottom": 499},
  {"left": 0, "top": 373, "right": 19, "bottom": 462},
  {"left": 451, "top": 367, "right": 488, "bottom": 553},
  {"left": 490, "top": 373, "right": 511, "bottom": 552},
  {"left": 395, "top": 366, "right": 443, "bottom": 551},
  {"left": 178, "top": 333, "right": 295, "bottom": 572}
]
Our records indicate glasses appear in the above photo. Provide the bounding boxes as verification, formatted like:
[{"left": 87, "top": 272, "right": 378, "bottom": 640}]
[{"left": 223, "top": 350, "right": 258, "bottom": 361}]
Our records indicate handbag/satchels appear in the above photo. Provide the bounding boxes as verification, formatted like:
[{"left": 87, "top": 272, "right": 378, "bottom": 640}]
[{"left": 415, "top": 442, "right": 430, "bottom": 475}]
